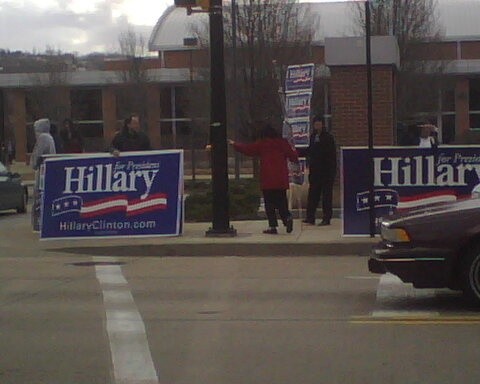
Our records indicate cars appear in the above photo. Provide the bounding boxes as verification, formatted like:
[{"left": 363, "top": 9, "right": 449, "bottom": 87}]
[
  {"left": 0, "top": 161, "right": 29, "bottom": 214},
  {"left": 368, "top": 197, "right": 480, "bottom": 311}
]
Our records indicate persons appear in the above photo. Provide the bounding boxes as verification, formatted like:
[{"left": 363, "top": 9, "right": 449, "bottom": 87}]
[
  {"left": 61, "top": 118, "right": 86, "bottom": 153},
  {"left": 106, "top": 114, "right": 154, "bottom": 158},
  {"left": 401, "top": 121, "right": 440, "bottom": 148},
  {"left": 301, "top": 115, "right": 336, "bottom": 226},
  {"left": 50, "top": 124, "right": 63, "bottom": 154},
  {"left": 226, "top": 125, "right": 299, "bottom": 235},
  {"left": 29, "top": 117, "right": 57, "bottom": 233}
]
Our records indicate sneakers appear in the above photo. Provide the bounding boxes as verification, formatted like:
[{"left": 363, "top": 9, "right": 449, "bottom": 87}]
[
  {"left": 262, "top": 228, "right": 278, "bottom": 234},
  {"left": 286, "top": 216, "right": 293, "bottom": 233}
]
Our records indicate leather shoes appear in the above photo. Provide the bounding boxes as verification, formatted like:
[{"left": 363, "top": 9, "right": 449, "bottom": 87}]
[
  {"left": 318, "top": 221, "right": 330, "bottom": 225},
  {"left": 301, "top": 219, "right": 315, "bottom": 224}
]
